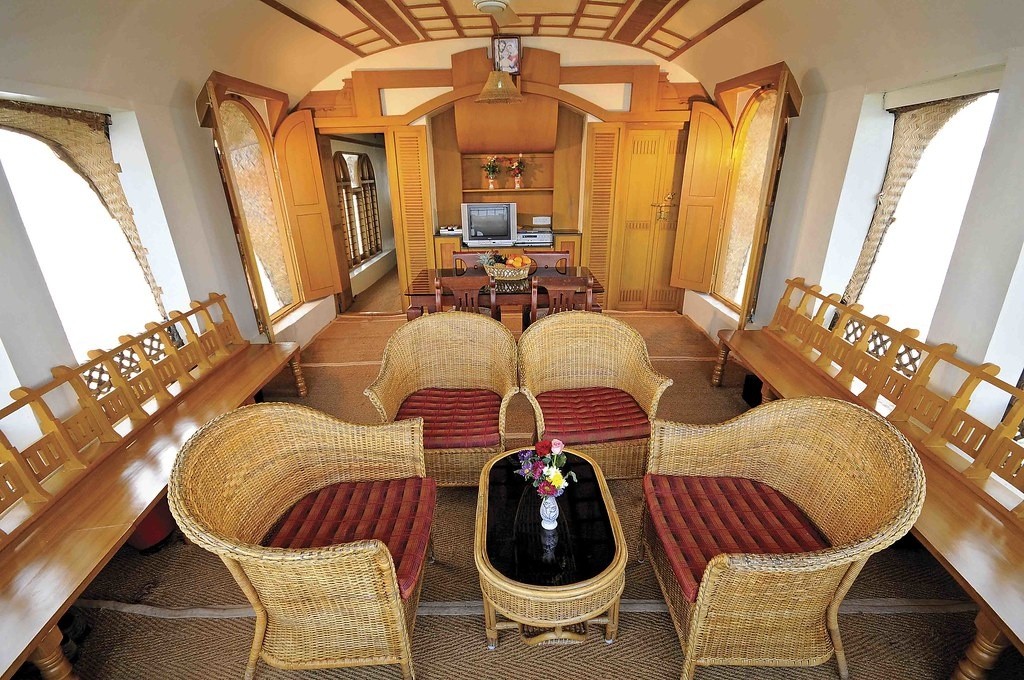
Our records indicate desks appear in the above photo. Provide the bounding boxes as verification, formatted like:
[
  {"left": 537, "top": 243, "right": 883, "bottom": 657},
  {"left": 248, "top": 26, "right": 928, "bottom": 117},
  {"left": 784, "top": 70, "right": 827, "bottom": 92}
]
[{"left": 403, "top": 266, "right": 604, "bottom": 322}]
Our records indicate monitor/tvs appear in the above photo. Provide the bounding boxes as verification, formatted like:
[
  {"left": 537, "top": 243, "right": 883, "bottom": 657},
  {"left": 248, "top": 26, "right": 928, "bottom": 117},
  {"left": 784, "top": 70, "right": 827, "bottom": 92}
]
[{"left": 461, "top": 202, "right": 517, "bottom": 247}]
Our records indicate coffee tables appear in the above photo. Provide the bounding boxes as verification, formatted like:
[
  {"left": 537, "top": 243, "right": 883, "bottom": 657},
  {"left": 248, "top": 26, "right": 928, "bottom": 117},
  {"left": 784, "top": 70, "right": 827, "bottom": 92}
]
[{"left": 474, "top": 446, "right": 628, "bottom": 650}]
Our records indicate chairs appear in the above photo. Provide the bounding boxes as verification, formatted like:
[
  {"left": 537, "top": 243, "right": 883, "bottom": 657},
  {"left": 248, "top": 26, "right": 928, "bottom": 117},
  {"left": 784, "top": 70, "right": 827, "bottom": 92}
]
[
  {"left": 434, "top": 250, "right": 594, "bottom": 334},
  {"left": 636, "top": 397, "right": 927, "bottom": 680},
  {"left": 363, "top": 311, "right": 674, "bottom": 487},
  {"left": 166, "top": 402, "right": 436, "bottom": 680}
]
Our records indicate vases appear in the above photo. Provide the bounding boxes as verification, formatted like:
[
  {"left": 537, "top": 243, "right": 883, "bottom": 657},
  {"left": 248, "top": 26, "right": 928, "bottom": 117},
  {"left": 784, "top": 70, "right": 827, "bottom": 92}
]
[
  {"left": 488, "top": 175, "right": 494, "bottom": 190},
  {"left": 515, "top": 173, "right": 521, "bottom": 189},
  {"left": 540, "top": 497, "right": 559, "bottom": 530}
]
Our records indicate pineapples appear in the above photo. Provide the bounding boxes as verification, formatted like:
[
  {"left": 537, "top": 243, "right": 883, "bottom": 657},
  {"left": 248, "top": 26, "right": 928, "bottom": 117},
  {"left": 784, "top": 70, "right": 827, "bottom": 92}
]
[{"left": 493, "top": 263, "right": 506, "bottom": 268}]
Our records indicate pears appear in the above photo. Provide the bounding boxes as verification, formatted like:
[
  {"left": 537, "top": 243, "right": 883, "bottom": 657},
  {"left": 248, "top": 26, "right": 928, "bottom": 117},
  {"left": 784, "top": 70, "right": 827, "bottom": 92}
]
[{"left": 506, "top": 255, "right": 531, "bottom": 268}]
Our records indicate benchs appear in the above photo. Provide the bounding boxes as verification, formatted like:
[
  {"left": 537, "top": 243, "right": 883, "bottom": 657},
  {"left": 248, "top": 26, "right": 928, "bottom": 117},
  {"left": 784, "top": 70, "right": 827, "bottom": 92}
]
[
  {"left": 711, "top": 277, "right": 1024, "bottom": 680},
  {"left": 0, "top": 293, "right": 307, "bottom": 680}
]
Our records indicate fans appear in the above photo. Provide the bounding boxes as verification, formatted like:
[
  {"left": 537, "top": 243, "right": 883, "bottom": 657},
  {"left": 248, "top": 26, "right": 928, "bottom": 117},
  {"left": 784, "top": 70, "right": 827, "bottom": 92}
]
[{"left": 472, "top": 0, "right": 521, "bottom": 28}]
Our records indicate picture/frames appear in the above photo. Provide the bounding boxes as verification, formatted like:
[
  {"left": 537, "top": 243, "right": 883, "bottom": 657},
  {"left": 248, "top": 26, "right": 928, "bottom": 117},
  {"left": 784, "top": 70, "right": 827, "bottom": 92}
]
[{"left": 492, "top": 36, "right": 522, "bottom": 76}]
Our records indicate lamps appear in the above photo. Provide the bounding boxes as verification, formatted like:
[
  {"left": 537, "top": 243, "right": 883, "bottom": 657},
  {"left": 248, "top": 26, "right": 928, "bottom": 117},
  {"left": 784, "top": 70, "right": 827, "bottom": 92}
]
[{"left": 473, "top": 26, "right": 523, "bottom": 105}]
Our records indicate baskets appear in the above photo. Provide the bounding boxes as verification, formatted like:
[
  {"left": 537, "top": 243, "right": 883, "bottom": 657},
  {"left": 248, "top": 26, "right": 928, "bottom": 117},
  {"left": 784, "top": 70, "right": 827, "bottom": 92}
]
[{"left": 483, "top": 254, "right": 531, "bottom": 280}]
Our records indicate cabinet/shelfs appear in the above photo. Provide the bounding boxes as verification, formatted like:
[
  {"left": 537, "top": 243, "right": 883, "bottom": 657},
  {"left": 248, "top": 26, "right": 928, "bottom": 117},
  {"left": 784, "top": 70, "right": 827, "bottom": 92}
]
[
  {"left": 460, "top": 153, "right": 554, "bottom": 192},
  {"left": 434, "top": 233, "right": 582, "bottom": 268}
]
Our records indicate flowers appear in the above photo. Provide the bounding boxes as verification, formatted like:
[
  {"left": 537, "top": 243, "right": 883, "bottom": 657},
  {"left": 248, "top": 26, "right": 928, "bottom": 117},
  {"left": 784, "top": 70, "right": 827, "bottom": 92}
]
[
  {"left": 480, "top": 153, "right": 528, "bottom": 177},
  {"left": 513, "top": 438, "right": 577, "bottom": 501}
]
